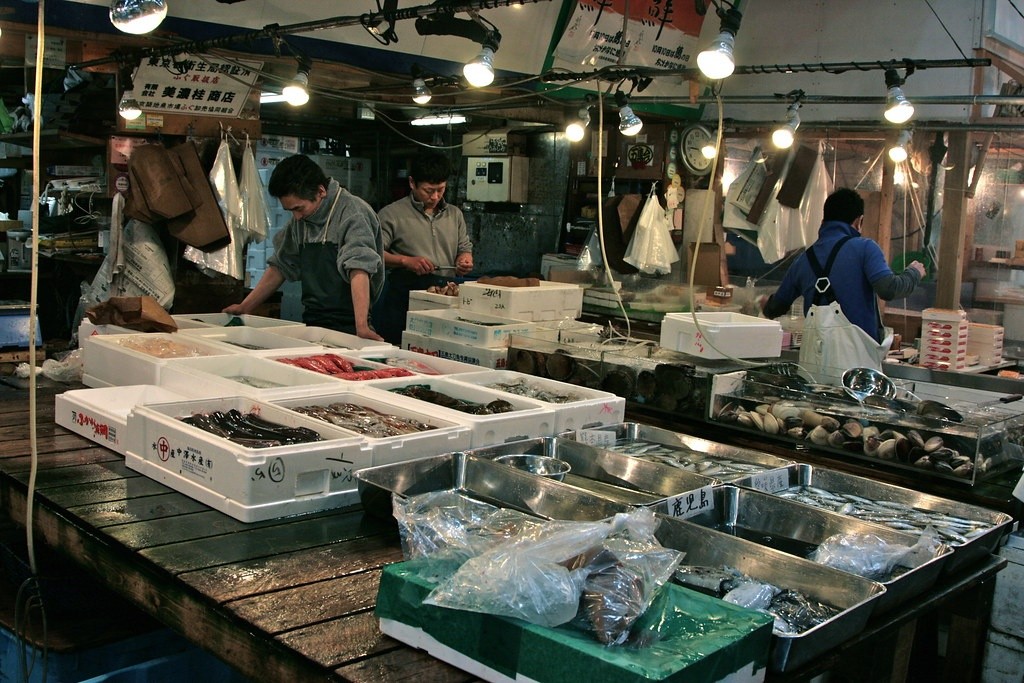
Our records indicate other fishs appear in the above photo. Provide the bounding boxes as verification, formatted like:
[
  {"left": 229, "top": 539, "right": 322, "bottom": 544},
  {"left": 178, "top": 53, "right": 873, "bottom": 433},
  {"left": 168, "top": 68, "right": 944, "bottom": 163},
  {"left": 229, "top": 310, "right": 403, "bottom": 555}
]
[
  {"left": 387, "top": 381, "right": 512, "bottom": 418},
  {"left": 282, "top": 354, "right": 413, "bottom": 381},
  {"left": 174, "top": 406, "right": 321, "bottom": 449},
  {"left": 572, "top": 545, "right": 646, "bottom": 647}
]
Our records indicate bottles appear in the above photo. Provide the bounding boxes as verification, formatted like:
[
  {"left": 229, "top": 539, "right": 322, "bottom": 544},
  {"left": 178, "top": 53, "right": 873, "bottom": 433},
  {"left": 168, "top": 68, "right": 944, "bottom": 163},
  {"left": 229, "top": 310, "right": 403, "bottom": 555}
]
[{"left": 975, "top": 248, "right": 983, "bottom": 260}]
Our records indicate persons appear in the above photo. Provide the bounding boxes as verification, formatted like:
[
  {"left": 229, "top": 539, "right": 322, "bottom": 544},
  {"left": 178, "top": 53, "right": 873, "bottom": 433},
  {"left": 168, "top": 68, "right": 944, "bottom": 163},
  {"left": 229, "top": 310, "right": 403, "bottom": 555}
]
[
  {"left": 754, "top": 186, "right": 927, "bottom": 347},
  {"left": 376, "top": 150, "right": 474, "bottom": 348},
  {"left": 222, "top": 155, "right": 386, "bottom": 343}
]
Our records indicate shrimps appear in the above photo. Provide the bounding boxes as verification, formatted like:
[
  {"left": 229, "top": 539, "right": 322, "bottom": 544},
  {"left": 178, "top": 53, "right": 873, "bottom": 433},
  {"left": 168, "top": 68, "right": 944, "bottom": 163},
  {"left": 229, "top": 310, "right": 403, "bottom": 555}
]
[{"left": 293, "top": 400, "right": 437, "bottom": 440}]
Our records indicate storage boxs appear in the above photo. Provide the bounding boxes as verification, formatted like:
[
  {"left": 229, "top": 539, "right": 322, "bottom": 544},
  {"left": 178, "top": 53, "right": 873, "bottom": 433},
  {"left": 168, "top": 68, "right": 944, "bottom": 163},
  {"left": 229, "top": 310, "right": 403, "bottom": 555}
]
[
  {"left": 967, "top": 323, "right": 1004, "bottom": 366},
  {"left": 660, "top": 311, "right": 783, "bottom": 360},
  {"left": 373, "top": 556, "right": 775, "bottom": 683},
  {"left": 0, "top": 622, "right": 245, "bottom": 683},
  {"left": 246, "top": 133, "right": 372, "bottom": 321},
  {"left": 585, "top": 123, "right": 665, "bottom": 179},
  {"left": 922, "top": 308, "right": 969, "bottom": 371},
  {"left": 402, "top": 254, "right": 600, "bottom": 369},
  {"left": 53, "top": 313, "right": 627, "bottom": 523},
  {"left": 462, "top": 128, "right": 527, "bottom": 156}
]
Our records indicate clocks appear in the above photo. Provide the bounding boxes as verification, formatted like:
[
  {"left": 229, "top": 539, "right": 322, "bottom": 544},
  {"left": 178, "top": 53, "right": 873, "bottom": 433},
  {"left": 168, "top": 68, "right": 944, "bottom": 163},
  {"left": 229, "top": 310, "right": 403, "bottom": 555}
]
[{"left": 682, "top": 126, "right": 713, "bottom": 176}]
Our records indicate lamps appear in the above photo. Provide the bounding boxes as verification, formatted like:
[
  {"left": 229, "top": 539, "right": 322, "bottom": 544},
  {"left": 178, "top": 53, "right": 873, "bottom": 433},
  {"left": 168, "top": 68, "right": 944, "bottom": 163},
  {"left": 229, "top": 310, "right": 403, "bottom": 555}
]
[
  {"left": 772, "top": 104, "right": 801, "bottom": 150},
  {"left": 109, "top": 0, "right": 169, "bottom": 35},
  {"left": 118, "top": 66, "right": 142, "bottom": 121},
  {"left": 697, "top": 8, "right": 742, "bottom": 81},
  {"left": 410, "top": 114, "right": 471, "bottom": 126},
  {"left": 615, "top": 90, "right": 643, "bottom": 136},
  {"left": 882, "top": 69, "right": 914, "bottom": 124},
  {"left": 410, "top": 64, "right": 432, "bottom": 104},
  {"left": 889, "top": 129, "right": 911, "bottom": 163},
  {"left": 564, "top": 108, "right": 591, "bottom": 142},
  {"left": 702, "top": 130, "right": 717, "bottom": 159},
  {"left": 282, "top": 57, "right": 313, "bottom": 107},
  {"left": 463, "top": 30, "right": 503, "bottom": 86}
]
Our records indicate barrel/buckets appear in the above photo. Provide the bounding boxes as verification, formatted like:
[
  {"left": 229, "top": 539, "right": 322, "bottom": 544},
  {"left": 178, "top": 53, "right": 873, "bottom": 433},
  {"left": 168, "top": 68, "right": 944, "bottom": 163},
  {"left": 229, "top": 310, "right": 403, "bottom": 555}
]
[{"left": 893, "top": 251, "right": 932, "bottom": 281}]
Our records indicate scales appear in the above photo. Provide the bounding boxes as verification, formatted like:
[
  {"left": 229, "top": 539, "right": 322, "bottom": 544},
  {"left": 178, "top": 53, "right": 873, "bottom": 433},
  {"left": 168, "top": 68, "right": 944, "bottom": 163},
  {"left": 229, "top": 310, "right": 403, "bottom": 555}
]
[{"left": 0, "top": 300, "right": 42, "bottom": 351}]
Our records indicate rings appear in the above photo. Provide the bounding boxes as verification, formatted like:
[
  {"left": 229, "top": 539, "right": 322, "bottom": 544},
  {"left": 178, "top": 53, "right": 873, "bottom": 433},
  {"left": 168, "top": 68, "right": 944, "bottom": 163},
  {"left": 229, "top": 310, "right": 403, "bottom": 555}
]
[
  {"left": 463, "top": 268, "right": 467, "bottom": 272},
  {"left": 419, "top": 266, "right": 424, "bottom": 272}
]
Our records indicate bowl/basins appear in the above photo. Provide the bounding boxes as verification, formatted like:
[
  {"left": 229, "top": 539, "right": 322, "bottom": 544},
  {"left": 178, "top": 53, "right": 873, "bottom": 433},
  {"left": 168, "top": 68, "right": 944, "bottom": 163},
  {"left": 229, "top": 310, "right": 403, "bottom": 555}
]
[
  {"left": 842, "top": 367, "right": 897, "bottom": 401},
  {"left": 492, "top": 454, "right": 572, "bottom": 482}
]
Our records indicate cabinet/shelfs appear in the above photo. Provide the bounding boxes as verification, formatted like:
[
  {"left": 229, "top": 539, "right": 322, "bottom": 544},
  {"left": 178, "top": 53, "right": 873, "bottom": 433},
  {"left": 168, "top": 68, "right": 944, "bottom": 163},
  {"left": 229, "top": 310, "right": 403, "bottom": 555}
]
[
  {"left": 0, "top": 129, "right": 152, "bottom": 288},
  {"left": 558, "top": 121, "right": 686, "bottom": 257}
]
